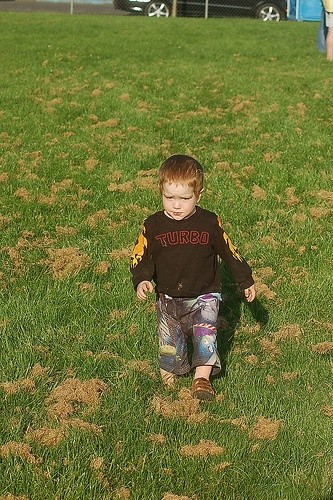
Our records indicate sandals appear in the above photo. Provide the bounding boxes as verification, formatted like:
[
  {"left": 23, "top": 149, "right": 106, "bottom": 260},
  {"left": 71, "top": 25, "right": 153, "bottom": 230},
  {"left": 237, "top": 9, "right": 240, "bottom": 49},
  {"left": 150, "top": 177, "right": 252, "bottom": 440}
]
[{"left": 190, "top": 378, "right": 216, "bottom": 403}]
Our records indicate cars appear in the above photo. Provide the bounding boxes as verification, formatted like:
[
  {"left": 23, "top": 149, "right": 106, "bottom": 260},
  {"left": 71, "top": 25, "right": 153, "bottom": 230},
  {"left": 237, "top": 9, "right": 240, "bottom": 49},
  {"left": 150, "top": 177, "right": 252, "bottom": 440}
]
[{"left": 113, "top": 0, "right": 286, "bottom": 22}]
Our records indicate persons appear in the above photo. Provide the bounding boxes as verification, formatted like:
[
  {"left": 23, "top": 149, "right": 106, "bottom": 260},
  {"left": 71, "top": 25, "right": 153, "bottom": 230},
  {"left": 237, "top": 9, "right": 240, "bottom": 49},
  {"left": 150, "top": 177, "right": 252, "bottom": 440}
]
[
  {"left": 321, "top": 0, "right": 333, "bottom": 60},
  {"left": 129, "top": 154, "right": 257, "bottom": 401}
]
